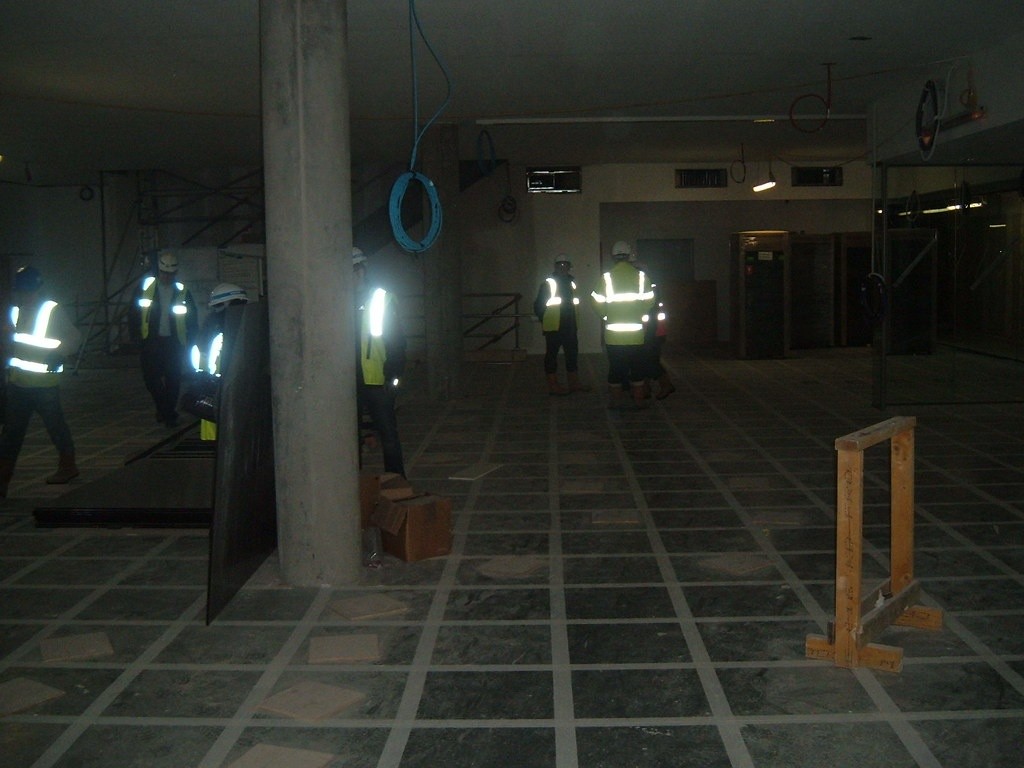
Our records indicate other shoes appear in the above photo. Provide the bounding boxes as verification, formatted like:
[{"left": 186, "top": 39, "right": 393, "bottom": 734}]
[{"left": 44, "top": 464, "right": 77, "bottom": 484}]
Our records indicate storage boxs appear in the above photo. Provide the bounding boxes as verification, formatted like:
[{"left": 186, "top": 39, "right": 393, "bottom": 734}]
[{"left": 359, "top": 468, "right": 450, "bottom": 563}]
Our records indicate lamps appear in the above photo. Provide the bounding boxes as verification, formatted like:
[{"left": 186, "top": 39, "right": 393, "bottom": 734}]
[{"left": 752, "top": 159, "right": 777, "bottom": 193}]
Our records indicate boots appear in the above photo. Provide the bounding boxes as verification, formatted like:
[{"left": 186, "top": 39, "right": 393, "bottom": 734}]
[
  {"left": 566, "top": 372, "right": 592, "bottom": 391},
  {"left": 652, "top": 371, "right": 676, "bottom": 400},
  {"left": 630, "top": 381, "right": 646, "bottom": 410},
  {"left": 607, "top": 383, "right": 632, "bottom": 411},
  {"left": 546, "top": 374, "right": 571, "bottom": 395}
]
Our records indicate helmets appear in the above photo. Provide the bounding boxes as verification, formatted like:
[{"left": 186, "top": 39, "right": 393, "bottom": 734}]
[
  {"left": 553, "top": 253, "right": 571, "bottom": 263},
  {"left": 12, "top": 267, "right": 45, "bottom": 294},
  {"left": 628, "top": 251, "right": 639, "bottom": 263},
  {"left": 207, "top": 282, "right": 251, "bottom": 307},
  {"left": 353, "top": 246, "right": 367, "bottom": 265},
  {"left": 159, "top": 254, "right": 183, "bottom": 271},
  {"left": 611, "top": 240, "right": 632, "bottom": 255}
]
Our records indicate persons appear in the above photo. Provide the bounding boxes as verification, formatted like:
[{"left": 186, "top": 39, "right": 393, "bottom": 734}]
[
  {"left": 0, "top": 265, "right": 81, "bottom": 501},
  {"left": 128, "top": 254, "right": 199, "bottom": 425},
  {"left": 353, "top": 247, "right": 406, "bottom": 478},
  {"left": 590, "top": 243, "right": 675, "bottom": 408},
  {"left": 533, "top": 253, "right": 582, "bottom": 394},
  {"left": 188, "top": 283, "right": 249, "bottom": 439}
]
